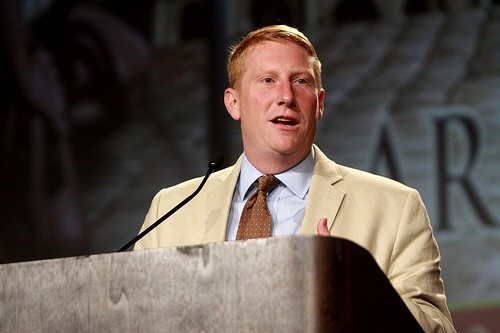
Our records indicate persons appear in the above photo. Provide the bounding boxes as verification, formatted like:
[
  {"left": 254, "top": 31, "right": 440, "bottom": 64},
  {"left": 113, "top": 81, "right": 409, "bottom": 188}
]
[{"left": 134, "top": 24, "right": 456, "bottom": 333}]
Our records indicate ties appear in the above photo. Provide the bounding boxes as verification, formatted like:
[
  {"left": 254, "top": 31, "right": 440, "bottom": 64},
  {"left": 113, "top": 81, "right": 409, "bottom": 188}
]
[{"left": 236, "top": 175, "right": 278, "bottom": 240}]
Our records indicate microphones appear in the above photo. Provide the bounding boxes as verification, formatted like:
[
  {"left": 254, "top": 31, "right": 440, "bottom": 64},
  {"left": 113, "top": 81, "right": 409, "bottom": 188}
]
[{"left": 117, "top": 155, "right": 224, "bottom": 252}]
